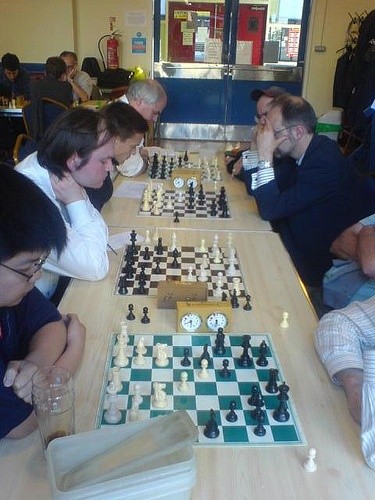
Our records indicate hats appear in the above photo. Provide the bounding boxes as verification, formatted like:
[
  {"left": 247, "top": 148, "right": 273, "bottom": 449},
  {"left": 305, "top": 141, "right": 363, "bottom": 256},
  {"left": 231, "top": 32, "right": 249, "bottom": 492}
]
[{"left": 251, "top": 86, "right": 287, "bottom": 102}]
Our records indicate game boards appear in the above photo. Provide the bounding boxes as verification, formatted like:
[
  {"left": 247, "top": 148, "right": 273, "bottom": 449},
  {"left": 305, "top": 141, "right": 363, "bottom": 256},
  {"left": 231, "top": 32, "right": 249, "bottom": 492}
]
[
  {"left": 112, "top": 243, "right": 250, "bottom": 300},
  {"left": 95, "top": 332, "right": 308, "bottom": 448},
  {"left": 136, "top": 186, "right": 234, "bottom": 220},
  {"left": 146, "top": 158, "right": 225, "bottom": 184}
]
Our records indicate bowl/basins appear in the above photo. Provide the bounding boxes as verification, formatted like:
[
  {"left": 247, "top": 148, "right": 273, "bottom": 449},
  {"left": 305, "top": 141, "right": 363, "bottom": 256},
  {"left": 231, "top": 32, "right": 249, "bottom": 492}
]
[{"left": 45, "top": 416, "right": 196, "bottom": 500}]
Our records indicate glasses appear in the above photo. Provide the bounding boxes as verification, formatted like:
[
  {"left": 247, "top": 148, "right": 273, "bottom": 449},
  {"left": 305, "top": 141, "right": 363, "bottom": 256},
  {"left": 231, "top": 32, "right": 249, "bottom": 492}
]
[
  {"left": 0, "top": 247, "right": 51, "bottom": 283},
  {"left": 273, "top": 125, "right": 297, "bottom": 139},
  {"left": 255, "top": 111, "right": 273, "bottom": 124},
  {"left": 66, "top": 66, "right": 74, "bottom": 70}
]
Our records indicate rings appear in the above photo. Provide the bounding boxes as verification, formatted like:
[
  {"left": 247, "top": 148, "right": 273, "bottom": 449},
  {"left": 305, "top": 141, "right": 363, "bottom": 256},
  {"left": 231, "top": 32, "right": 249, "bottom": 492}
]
[
  {"left": 227, "top": 156, "right": 230, "bottom": 159},
  {"left": 232, "top": 169, "right": 236, "bottom": 172}
]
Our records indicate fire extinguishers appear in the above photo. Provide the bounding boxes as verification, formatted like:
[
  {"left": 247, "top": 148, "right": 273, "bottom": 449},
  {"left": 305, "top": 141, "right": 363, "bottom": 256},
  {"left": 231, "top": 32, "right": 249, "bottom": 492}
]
[{"left": 98, "top": 30, "right": 122, "bottom": 71}]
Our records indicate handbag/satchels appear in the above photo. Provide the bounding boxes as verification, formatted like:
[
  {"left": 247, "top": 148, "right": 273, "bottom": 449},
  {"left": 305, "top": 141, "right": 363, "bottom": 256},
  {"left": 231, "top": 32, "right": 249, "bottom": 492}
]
[{"left": 81, "top": 57, "right": 102, "bottom": 77}]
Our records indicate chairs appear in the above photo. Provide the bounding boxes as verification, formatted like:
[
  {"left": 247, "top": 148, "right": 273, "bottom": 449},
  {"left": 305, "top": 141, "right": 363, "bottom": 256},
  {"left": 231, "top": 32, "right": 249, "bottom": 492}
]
[{"left": 12, "top": 98, "right": 68, "bottom": 166}]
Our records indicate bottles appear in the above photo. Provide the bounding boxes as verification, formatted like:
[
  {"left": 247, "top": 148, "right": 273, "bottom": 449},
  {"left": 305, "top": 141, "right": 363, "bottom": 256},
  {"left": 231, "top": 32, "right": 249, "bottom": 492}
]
[{"left": 30, "top": 365, "right": 75, "bottom": 454}]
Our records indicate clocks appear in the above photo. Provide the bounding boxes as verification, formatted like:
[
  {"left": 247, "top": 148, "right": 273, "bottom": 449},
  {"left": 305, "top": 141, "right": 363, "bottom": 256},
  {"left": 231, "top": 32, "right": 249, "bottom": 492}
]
[
  {"left": 176, "top": 302, "right": 232, "bottom": 334},
  {"left": 187, "top": 177, "right": 199, "bottom": 189},
  {"left": 173, "top": 176, "right": 185, "bottom": 189}
]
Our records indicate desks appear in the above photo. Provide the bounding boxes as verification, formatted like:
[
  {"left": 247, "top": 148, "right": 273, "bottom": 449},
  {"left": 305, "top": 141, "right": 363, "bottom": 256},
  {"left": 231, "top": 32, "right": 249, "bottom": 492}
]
[
  {"left": 0, "top": 99, "right": 154, "bottom": 147},
  {"left": 0, "top": 149, "right": 375, "bottom": 500}
]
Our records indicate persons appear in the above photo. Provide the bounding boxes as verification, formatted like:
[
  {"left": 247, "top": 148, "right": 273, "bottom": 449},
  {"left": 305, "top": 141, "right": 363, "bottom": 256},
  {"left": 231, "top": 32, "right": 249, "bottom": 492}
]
[
  {"left": 313, "top": 296, "right": 375, "bottom": 470},
  {"left": 60, "top": 51, "right": 93, "bottom": 104},
  {"left": 225, "top": 86, "right": 290, "bottom": 181},
  {"left": 306, "top": 212, "right": 375, "bottom": 321},
  {"left": 84, "top": 100, "right": 149, "bottom": 212},
  {"left": 24, "top": 56, "right": 73, "bottom": 108},
  {"left": 108, "top": 79, "right": 166, "bottom": 177},
  {"left": 0, "top": 52, "right": 31, "bottom": 164},
  {"left": 242, "top": 94, "right": 364, "bottom": 288},
  {"left": 0, "top": 164, "right": 85, "bottom": 442},
  {"left": 14, "top": 108, "right": 120, "bottom": 298}
]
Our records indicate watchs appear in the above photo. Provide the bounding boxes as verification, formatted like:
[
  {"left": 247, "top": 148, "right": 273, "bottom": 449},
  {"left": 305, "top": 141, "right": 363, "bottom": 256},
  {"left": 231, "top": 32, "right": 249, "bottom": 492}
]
[{"left": 257, "top": 161, "right": 273, "bottom": 170}]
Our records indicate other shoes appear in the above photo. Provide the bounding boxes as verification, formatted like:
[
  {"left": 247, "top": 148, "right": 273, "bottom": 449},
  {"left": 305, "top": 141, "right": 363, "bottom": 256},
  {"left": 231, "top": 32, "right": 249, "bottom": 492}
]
[{"left": 96, "top": 67, "right": 134, "bottom": 89}]
